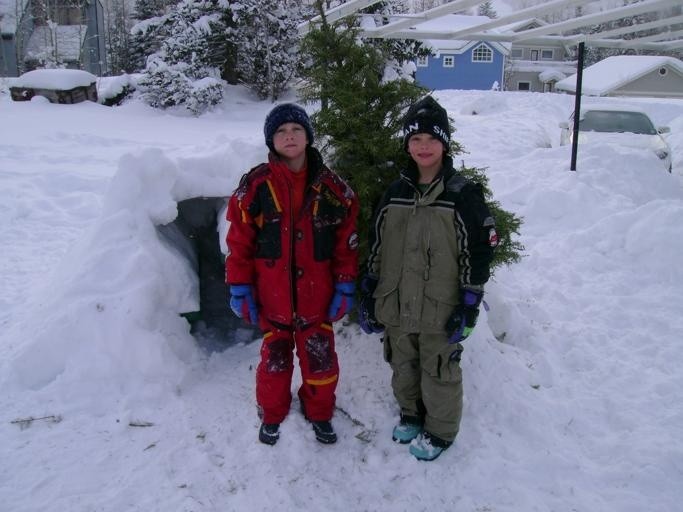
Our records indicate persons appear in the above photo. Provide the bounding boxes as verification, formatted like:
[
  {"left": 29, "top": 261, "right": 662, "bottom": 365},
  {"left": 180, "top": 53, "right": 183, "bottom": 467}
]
[
  {"left": 225, "top": 103, "right": 360, "bottom": 445},
  {"left": 359, "top": 96, "right": 498, "bottom": 461}
]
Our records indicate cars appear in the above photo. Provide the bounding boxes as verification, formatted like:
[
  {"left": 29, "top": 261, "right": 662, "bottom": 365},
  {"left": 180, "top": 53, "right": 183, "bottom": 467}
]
[{"left": 559, "top": 105, "right": 672, "bottom": 173}]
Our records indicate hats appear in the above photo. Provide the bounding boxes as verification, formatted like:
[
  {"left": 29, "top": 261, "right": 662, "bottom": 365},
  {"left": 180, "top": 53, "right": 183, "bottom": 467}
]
[
  {"left": 402, "top": 95, "right": 451, "bottom": 157},
  {"left": 263, "top": 102, "right": 315, "bottom": 153}
]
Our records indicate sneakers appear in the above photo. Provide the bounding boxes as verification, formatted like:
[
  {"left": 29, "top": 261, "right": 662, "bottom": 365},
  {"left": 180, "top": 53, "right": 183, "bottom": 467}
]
[
  {"left": 258, "top": 423, "right": 281, "bottom": 445},
  {"left": 304, "top": 415, "right": 338, "bottom": 444},
  {"left": 408, "top": 433, "right": 445, "bottom": 462},
  {"left": 391, "top": 421, "right": 422, "bottom": 445}
]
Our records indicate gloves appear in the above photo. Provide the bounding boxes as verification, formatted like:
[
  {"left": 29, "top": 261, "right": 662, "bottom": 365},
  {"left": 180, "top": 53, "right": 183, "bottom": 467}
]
[
  {"left": 228, "top": 284, "right": 258, "bottom": 327},
  {"left": 358, "top": 276, "right": 385, "bottom": 335},
  {"left": 326, "top": 280, "right": 358, "bottom": 323},
  {"left": 444, "top": 288, "right": 490, "bottom": 344}
]
